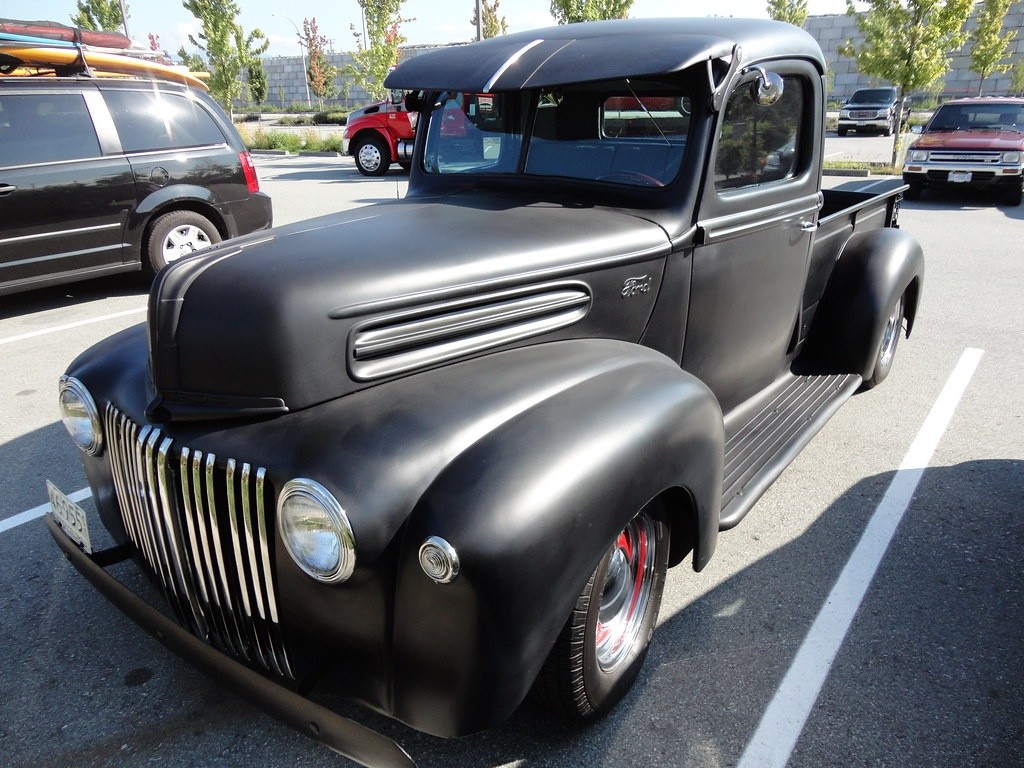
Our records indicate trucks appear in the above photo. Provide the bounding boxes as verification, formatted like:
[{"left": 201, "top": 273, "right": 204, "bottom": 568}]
[{"left": 343, "top": 63, "right": 683, "bottom": 178}]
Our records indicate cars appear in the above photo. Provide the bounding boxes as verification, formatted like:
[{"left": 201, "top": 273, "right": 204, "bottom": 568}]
[{"left": 902, "top": 91, "right": 1024, "bottom": 207}]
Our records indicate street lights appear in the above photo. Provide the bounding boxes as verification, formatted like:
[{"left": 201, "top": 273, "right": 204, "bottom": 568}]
[{"left": 272, "top": 14, "right": 310, "bottom": 107}]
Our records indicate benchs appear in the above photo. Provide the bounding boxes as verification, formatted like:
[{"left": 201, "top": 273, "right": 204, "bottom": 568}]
[{"left": 525, "top": 140, "right": 686, "bottom": 185}]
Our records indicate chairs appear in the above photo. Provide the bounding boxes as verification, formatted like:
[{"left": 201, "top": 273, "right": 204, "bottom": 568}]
[{"left": 999, "top": 113, "right": 1017, "bottom": 126}]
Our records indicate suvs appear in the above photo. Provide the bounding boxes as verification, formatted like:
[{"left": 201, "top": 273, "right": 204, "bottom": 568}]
[
  {"left": 837, "top": 86, "right": 913, "bottom": 137},
  {"left": 0, "top": 76, "right": 274, "bottom": 299}
]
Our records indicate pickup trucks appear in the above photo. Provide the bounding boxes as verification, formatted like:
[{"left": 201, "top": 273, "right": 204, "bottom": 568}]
[{"left": 42, "top": 10, "right": 928, "bottom": 739}]
